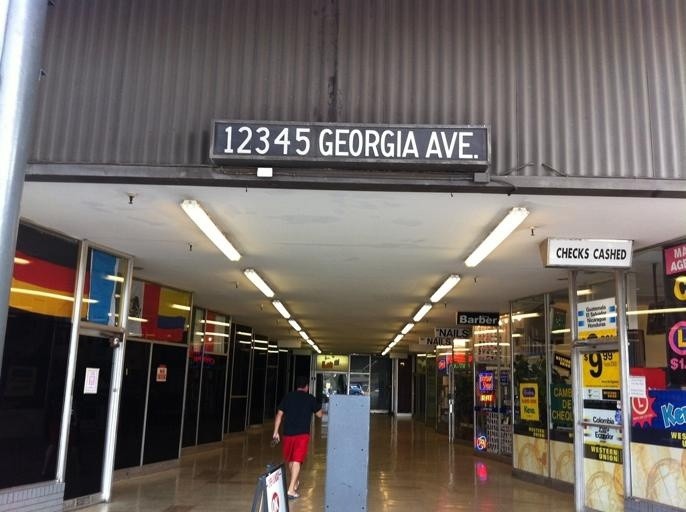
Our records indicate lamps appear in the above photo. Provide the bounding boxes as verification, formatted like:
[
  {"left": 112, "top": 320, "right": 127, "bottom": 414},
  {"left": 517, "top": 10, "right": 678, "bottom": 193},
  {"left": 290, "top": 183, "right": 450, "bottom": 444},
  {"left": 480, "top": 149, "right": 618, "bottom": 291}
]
[
  {"left": 381, "top": 303, "right": 432, "bottom": 356},
  {"left": 244, "top": 269, "right": 274, "bottom": 299},
  {"left": 180, "top": 199, "right": 242, "bottom": 263},
  {"left": 465, "top": 207, "right": 530, "bottom": 268},
  {"left": 429, "top": 273, "right": 461, "bottom": 304},
  {"left": 272, "top": 300, "right": 322, "bottom": 354}
]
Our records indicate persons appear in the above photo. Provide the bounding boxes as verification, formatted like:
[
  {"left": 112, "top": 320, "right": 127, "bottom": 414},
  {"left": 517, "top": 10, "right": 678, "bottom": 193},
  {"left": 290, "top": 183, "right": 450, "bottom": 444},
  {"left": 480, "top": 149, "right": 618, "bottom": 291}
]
[{"left": 271, "top": 375, "right": 322, "bottom": 498}]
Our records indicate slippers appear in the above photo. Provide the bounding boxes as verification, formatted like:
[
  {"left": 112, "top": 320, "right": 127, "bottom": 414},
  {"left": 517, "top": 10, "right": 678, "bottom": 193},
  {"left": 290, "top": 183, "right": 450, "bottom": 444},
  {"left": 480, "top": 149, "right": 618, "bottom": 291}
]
[{"left": 287, "top": 479, "right": 301, "bottom": 499}]
